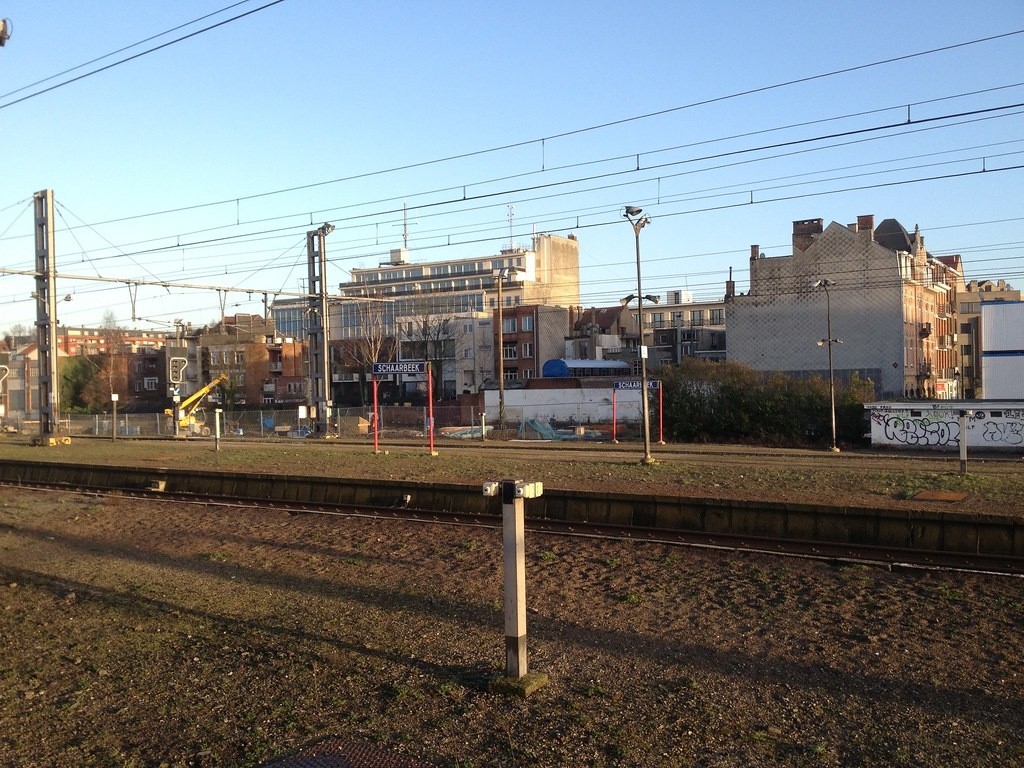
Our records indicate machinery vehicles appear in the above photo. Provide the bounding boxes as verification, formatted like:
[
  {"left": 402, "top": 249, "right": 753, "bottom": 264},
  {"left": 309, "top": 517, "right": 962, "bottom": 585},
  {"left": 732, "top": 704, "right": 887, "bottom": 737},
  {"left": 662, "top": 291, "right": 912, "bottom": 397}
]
[{"left": 164, "top": 374, "right": 230, "bottom": 437}]
